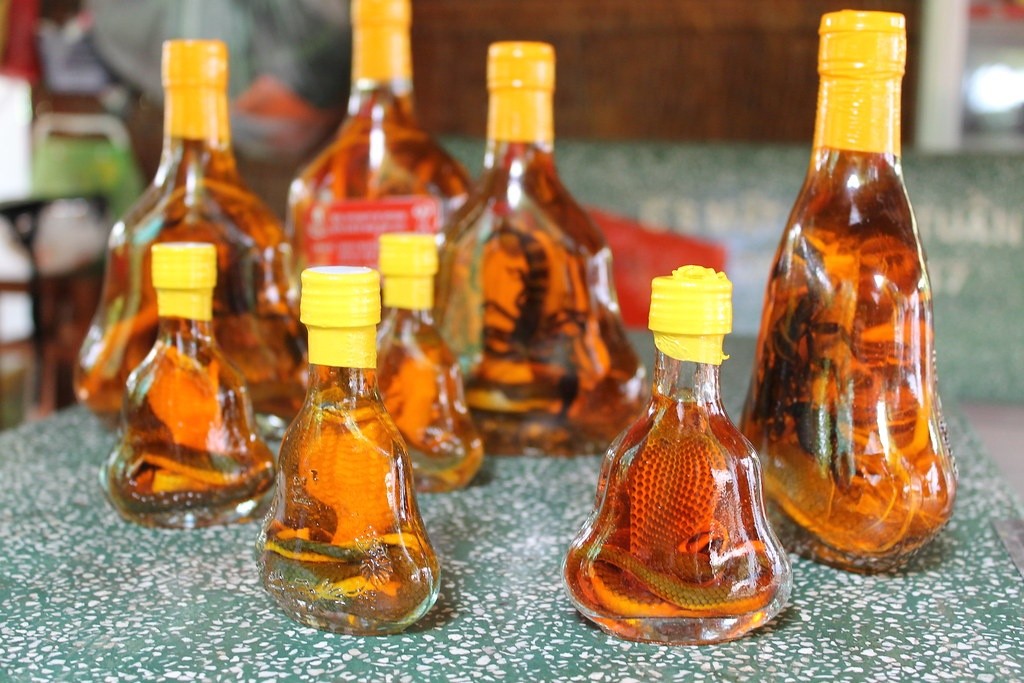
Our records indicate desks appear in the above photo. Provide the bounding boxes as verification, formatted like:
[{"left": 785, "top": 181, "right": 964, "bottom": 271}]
[{"left": 1, "top": 331, "right": 1022, "bottom": 683}]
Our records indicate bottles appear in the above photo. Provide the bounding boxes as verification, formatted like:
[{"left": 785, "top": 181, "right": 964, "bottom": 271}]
[
  {"left": 97, "top": 239, "right": 278, "bottom": 529},
  {"left": 257, "top": 265, "right": 440, "bottom": 636},
  {"left": 287, "top": 0, "right": 476, "bottom": 326},
  {"left": 437, "top": 41, "right": 648, "bottom": 459},
  {"left": 561, "top": 264, "right": 797, "bottom": 648},
  {"left": 375, "top": 233, "right": 484, "bottom": 492},
  {"left": 74, "top": 39, "right": 307, "bottom": 442},
  {"left": 738, "top": 11, "right": 957, "bottom": 577}
]
